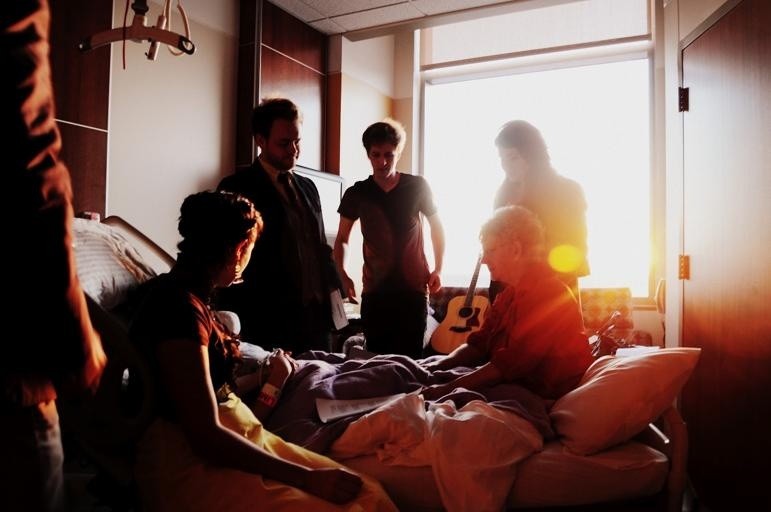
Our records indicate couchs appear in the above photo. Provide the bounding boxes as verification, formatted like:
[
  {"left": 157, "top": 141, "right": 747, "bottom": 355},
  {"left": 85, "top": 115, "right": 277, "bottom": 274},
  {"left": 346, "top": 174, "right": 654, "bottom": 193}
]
[{"left": 423, "top": 280, "right": 656, "bottom": 358}]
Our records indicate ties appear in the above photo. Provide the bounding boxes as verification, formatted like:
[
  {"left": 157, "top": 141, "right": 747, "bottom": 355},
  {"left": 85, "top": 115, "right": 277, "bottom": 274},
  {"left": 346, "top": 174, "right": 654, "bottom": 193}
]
[{"left": 276, "top": 171, "right": 303, "bottom": 218}]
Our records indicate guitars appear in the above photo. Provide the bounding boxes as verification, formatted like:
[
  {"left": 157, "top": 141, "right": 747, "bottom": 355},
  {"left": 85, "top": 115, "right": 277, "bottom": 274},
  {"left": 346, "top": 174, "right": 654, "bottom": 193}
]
[{"left": 432, "top": 255, "right": 491, "bottom": 354}]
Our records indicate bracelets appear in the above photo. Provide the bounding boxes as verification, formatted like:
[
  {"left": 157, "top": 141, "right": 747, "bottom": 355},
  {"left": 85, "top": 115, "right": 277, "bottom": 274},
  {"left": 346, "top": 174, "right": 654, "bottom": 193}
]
[{"left": 259, "top": 382, "right": 280, "bottom": 407}]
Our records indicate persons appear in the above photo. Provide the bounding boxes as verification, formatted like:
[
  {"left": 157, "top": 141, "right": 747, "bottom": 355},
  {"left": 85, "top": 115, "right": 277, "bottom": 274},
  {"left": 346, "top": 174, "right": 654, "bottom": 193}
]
[
  {"left": 257, "top": 350, "right": 294, "bottom": 420},
  {"left": 486, "top": 119, "right": 585, "bottom": 300},
  {"left": 421, "top": 205, "right": 593, "bottom": 400},
  {"left": 335, "top": 122, "right": 444, "bottom": 358},
  {"left": 0, "top": 0, "right": 108, "bottom": 509},
  {"left": 217, "top": 98, "right": 342, "bottom": 354},
  {"left": 113, "top": 191, "right": 392, "bottom": 510}
]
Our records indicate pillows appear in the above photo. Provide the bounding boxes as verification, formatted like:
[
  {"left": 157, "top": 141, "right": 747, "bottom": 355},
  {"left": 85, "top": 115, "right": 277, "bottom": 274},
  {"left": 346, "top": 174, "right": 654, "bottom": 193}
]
[{"left": 550, "top": 342, "right": 706, "bottom": 450}]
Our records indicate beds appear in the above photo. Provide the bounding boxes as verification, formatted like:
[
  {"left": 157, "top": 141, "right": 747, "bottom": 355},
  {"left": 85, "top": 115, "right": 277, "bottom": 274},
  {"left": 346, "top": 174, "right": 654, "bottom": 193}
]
[{"left": 62, "top": 212, "right": 695, "bottom": 511}]
